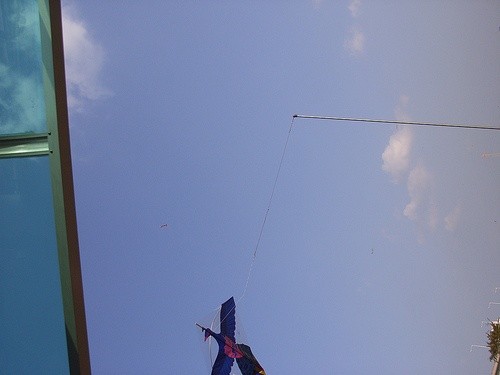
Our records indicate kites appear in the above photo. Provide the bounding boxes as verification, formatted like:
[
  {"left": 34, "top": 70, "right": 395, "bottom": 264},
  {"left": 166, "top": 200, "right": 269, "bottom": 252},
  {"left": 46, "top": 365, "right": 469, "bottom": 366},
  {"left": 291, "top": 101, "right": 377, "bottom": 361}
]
[{"left": 197, "top": 296, "right": 268, "bottom": 374}]
